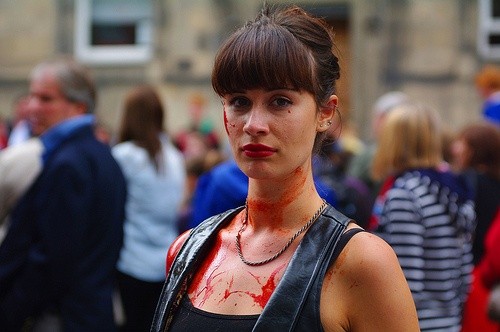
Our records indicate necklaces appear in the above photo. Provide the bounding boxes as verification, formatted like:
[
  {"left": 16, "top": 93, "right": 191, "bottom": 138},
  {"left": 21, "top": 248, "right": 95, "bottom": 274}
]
[{"left": 235, "top": 198, "right": 326, "bottom": 266}]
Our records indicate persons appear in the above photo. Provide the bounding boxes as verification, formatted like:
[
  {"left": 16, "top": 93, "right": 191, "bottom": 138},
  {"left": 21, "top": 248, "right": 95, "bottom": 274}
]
[
  {"left": 173, "top": 65, "right": 500, "bottom": 332},
  {"left": 110, "top": 86, "right": 188, "bottom": 332},
  {"left": 149, "top": 8, "right": 420, "bottom": 332},
  {"left": 0, "top": 95, "right": 108, "bottom": 243},
  {"left": 0, "top": 62, "right": 129, "bottom": 332}
]
